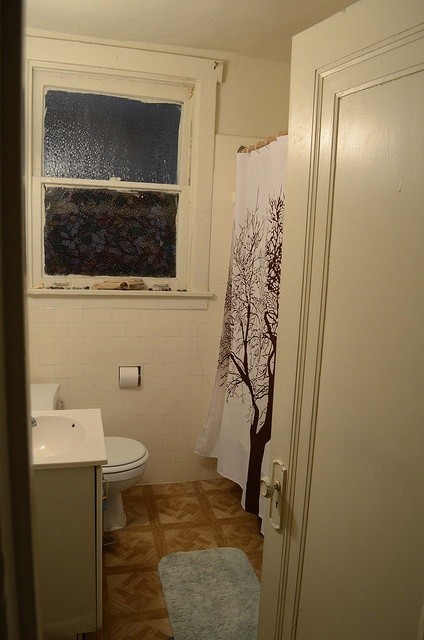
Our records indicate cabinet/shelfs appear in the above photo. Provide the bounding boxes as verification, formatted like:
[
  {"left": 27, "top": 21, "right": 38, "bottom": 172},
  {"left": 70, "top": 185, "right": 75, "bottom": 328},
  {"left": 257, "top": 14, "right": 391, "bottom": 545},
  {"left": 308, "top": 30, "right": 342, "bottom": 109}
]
[{"left": 30, "top": 464, "right": 109, "bottom": 640}]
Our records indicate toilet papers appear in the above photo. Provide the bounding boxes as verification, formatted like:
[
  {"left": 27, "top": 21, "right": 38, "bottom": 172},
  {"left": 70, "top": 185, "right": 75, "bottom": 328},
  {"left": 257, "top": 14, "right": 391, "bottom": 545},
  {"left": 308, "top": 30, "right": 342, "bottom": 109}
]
[{"left": 115, "top": 363, "right": 145, "bottom": 391}]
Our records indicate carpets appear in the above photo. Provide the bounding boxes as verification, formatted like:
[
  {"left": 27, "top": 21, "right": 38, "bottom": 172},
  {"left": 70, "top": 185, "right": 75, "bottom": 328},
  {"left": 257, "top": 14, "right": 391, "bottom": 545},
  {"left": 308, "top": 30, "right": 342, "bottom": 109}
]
[{"left": 158, "top": 547, "right": 260, "bottom": 639}]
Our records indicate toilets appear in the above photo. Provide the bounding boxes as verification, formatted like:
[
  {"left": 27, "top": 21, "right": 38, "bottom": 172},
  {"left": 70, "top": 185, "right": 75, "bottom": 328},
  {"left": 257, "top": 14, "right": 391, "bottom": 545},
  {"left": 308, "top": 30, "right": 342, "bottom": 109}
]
[{"left": 29, "top": 382, "right": 150, "bottom": 533}]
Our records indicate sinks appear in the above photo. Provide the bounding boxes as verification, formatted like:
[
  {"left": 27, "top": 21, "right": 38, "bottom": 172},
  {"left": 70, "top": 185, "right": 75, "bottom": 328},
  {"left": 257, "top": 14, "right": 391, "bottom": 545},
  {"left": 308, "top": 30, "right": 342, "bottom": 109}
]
[{"left": 27, "top": 408, "right": 107, "bottom": 464}]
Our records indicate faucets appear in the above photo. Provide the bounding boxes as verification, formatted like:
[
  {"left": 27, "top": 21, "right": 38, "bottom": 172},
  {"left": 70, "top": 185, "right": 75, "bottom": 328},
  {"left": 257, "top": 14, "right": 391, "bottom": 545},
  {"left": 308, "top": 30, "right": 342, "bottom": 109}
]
[{"left": 29, "top": 416, "right": 37, "bottom": 428}]
